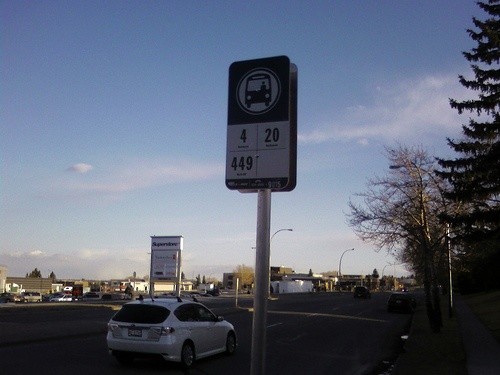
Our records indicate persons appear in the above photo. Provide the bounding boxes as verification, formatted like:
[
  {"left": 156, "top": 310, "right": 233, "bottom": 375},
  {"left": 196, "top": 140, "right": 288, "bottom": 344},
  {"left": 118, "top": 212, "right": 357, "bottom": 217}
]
[{"left": 127, "top": 283, "right": 134, "bottom": 296}]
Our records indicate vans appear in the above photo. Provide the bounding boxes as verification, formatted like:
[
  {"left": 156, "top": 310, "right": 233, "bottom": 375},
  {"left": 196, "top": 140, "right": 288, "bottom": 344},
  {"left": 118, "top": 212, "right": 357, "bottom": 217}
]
[{"left": 19, "top": 292, "right": 43, "bottom": 303}]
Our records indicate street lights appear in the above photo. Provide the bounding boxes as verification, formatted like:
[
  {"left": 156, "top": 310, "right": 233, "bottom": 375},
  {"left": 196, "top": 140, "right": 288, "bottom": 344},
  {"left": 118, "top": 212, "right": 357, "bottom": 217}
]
[
  {"left": 390, "top": 164, "right": 455, "bottom": 316},
  {"left": 339, "top": 248, "right": 354, "bottom": 291}
]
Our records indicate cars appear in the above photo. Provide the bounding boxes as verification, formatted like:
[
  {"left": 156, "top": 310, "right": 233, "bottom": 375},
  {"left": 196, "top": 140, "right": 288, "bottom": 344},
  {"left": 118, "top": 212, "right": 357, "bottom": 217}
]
[
  {"left": 199, "top": 288, "right": 222, "bottom": 296},
  {"left": 106, "top": 298, "right": 238, "bottom": 368},
  {"left": 387, "top": 293, "right": 415, "bottom": 313},
  {"left": 0, "top": 293, "right": 25, "bottom": 303},
  {"left": 47, "top": 292, "right": 103, "bottom": 303},
  {"left": 353, "top": 286, "right": 371, "bottom": 299}
]
